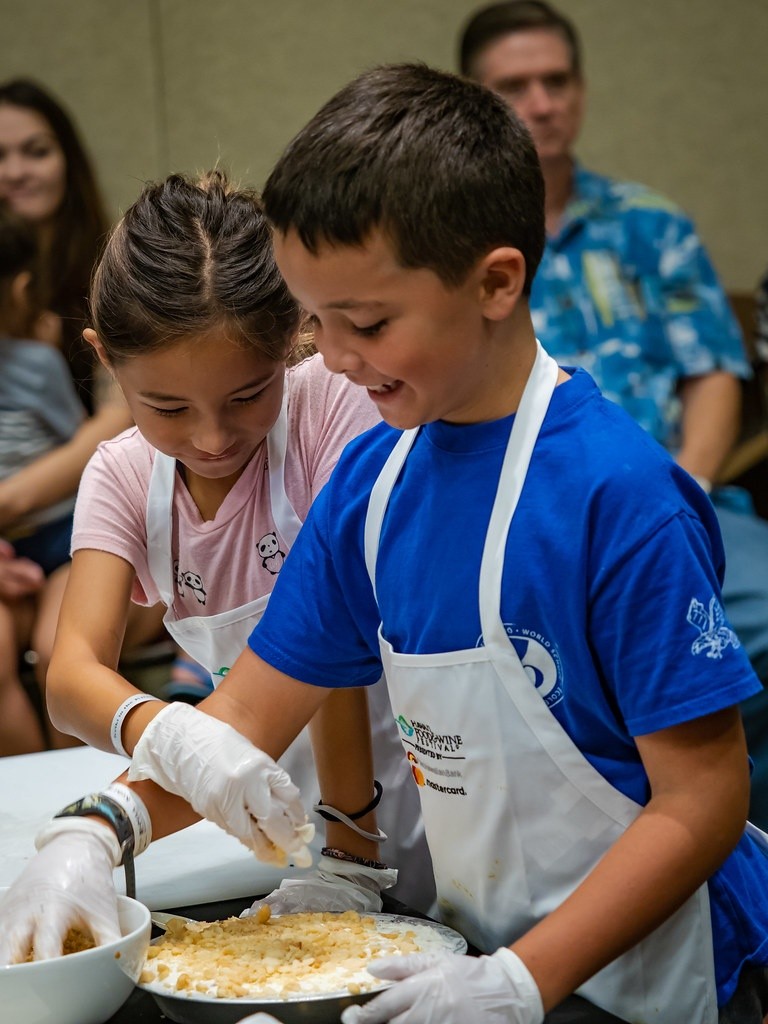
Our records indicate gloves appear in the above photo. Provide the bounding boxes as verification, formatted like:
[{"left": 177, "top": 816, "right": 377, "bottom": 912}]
[
  {"left": 237, "top": 858, "right": 399, "bottom": 913},
  {"left": 340, "top": 945, "right": 544, "bottom": 1024},
  {"left": 129, "top": 702, "right": 314, "bottom": 869},
  {"left": 0, "top": 815, "right": 122, "bottom": 965}
]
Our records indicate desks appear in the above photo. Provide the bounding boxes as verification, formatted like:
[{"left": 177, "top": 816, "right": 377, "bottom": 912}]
[{"left": 0, "top": 745, "right": 630, "bottom": 1024}]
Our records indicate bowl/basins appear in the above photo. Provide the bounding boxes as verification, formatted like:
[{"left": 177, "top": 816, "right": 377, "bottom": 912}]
[
  {"left": 138, "top": 911, "right": 469, "bottom": 1024},
  {"left": 0, "top": 884, "right": 152, "bottom": 1024}
]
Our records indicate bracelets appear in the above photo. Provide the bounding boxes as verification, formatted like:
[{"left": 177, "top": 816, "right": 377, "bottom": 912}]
[
  {"left": 313, "top": 780, "right": 387, "bottom": 843},
  {"left": 98, "top": 782, "right": 152, "bottom": 857},
  {"left": 320, "top": 847, "right": 387, "bottom": 870},
  {"left": 110, "top": 694, "right": 161, "bottom": 758}
]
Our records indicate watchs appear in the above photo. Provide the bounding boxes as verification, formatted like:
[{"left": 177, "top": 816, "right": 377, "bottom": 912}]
[{"left": 53, "top": 793, "right": 136, "bottom": 899}]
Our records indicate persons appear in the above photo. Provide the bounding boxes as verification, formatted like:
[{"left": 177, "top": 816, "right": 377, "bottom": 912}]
[
  {"left": 46, "top": 169, "right": 437, "bottom": 917},
  {"left": 0, "top": 72, "right": 136, "bottom": 757},
  {"left": 0, "top": 64, "right": 768, "bottom": 1024},
  {"left": 459, "top": 0, "right": 768, "bottom": 711}
]
